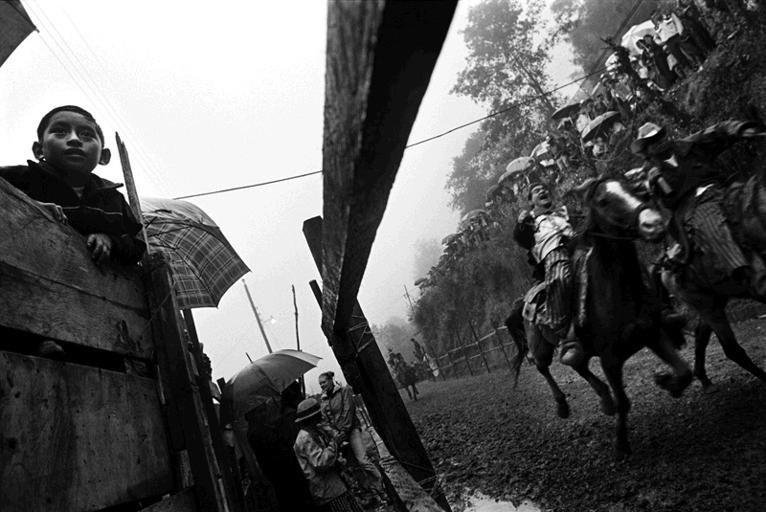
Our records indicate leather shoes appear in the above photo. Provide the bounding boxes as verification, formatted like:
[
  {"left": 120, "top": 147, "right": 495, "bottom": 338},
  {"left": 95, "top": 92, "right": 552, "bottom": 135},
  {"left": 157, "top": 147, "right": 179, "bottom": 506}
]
[{"left": 561, "top": 345, "right": 582, "bottom": 364}]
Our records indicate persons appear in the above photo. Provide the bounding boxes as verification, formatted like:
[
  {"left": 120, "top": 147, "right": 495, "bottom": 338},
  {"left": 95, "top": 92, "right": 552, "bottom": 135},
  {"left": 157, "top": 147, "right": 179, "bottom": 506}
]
[
  {"left": 243, "top": 371, "right": 386, "bottom": 512},
  {"left": 388, "top": 349, "right": 406, "bottom": 374},
  {"left": 0, "top": 104, "right": 146, "bottom": 358},
  {"left": 411, "top": 0, "right": 766, "bottom": 366}
]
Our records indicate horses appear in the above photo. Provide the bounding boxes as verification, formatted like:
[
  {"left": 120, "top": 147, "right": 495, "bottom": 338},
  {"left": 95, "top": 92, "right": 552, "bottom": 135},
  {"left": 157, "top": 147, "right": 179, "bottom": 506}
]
[
  {"left": 396, "top": 360, "right": 420, "bottom": 401},
  {"left": 504, "top": 171, "right": 696, "bottom": 462},
  {"left": 651, "top": 176, "right": 766, "bottom": 390}
]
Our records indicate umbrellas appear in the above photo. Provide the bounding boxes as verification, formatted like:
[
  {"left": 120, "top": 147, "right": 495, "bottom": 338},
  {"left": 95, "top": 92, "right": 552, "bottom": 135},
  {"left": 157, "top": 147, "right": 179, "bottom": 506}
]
[
  {"left": 219, "top": 348, "right": 323, "bottom": 426},
  {"left": 142, "top": 199, "right": 253, "bottom": 310}
]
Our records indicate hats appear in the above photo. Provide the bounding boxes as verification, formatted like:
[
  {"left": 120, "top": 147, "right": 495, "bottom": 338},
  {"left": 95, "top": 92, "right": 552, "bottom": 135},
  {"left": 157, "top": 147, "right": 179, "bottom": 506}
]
[
  {"left": 631, "top": 122, "right": 667, "bottom": 157},
  {"left": 294, "top": 397, "right": 328, "bottom": 423}
]
